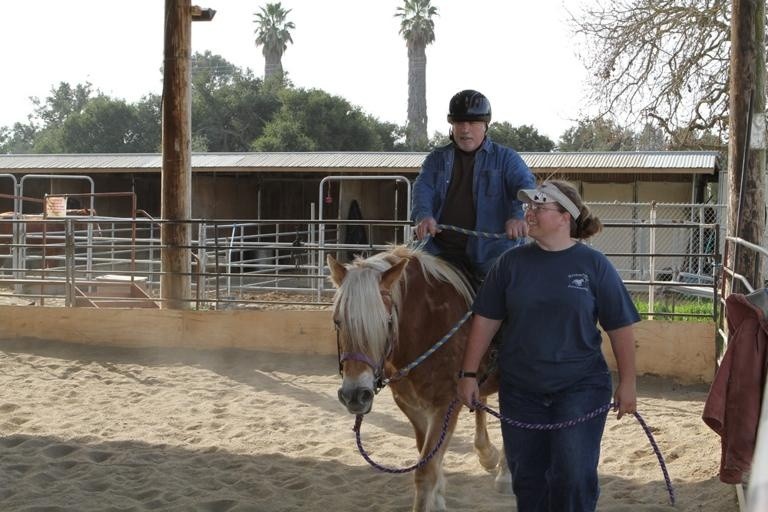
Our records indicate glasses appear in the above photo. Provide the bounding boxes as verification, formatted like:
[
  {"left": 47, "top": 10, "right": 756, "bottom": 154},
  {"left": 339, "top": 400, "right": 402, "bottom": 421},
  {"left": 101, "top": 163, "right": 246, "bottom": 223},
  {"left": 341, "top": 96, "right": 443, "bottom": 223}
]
[{"left": 522, "top": 203, "right": 559, "bottom": 212}]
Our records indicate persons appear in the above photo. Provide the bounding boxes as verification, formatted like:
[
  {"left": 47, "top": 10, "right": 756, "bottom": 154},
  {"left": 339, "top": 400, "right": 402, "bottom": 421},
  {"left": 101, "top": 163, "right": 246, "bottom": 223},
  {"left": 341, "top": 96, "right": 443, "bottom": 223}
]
[
  {"left": 409, "top": 89, "right": 537, "bottom": 303},
  {"left": 457, "top": 179, "right": 642, "bottom": 512}
]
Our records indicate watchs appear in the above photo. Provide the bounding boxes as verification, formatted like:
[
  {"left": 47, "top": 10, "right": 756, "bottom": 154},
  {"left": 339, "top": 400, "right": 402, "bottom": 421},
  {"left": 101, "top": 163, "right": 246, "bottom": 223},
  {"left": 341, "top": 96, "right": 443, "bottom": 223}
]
[{"left": 457, "top": 368, "right": 477, "bottom": 379}]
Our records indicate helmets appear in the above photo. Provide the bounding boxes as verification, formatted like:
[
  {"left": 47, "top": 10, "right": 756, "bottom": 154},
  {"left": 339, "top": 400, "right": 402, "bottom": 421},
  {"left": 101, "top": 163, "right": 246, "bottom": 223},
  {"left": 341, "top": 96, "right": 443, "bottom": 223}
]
[{"left": 448, "top": 90, "right": 491, "bottom": 122}]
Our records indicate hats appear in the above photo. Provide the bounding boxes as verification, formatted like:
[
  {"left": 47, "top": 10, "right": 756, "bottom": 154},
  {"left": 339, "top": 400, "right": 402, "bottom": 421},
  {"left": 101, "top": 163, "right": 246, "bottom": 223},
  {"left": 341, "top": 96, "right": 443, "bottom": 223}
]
[{"left": 517, "top": 182, "right": 580, "bottom": 220}]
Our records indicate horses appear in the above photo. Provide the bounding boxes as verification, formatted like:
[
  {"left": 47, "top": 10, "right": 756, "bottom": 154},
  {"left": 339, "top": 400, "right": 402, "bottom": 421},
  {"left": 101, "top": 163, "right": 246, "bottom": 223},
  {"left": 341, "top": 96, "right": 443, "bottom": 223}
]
[
  {"left": 326, "top": 241, "right": 514, "bottom": 512},
  {"left": 0, "top": 208, "right": 103, "bottom": 292}
]
